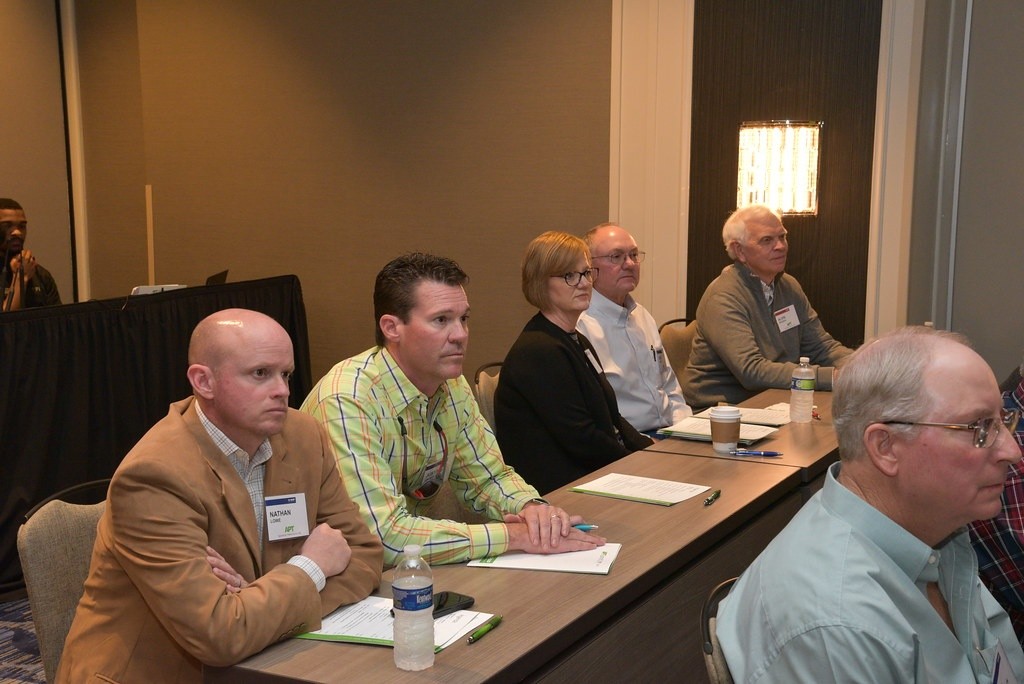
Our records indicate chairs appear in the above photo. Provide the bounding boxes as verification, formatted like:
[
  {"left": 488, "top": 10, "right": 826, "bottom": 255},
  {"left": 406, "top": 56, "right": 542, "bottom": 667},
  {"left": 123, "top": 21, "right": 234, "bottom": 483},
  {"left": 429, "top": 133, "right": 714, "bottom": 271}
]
[
  {"left": 472, "top": 356, "right": 510, "bottom": 431},
  {"left": 18, "top": 478, "right": 113, "bottom": 681},
  {"left": 700, "top": 574, "right": 741, "bottom": 684},
  {"left": 659, "top": 316, "right": 704, "bottom": 398}
]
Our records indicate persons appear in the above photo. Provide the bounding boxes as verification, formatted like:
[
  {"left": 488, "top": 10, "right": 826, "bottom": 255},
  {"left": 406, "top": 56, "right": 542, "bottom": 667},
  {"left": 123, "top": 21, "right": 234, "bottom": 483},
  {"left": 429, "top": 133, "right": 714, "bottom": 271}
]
[
  {"left": 299, "top": 253, "right": 607, "bottom": 571},
  {"left": 716, "top": 325, "right": 1024, "bottom": 684},
  {"left": 681, "top": 203, "right": 856, "bottom": 414},
  {"left": 55, "top": 309, "right": 386, "bottom": 684},
  {"left": 0, "top": 198, "right": 62, "bottom": 311},
  {"left": 966, "top": 361, "right": 1024, "bottom": 649},
  {"left": 494, "top": 230, "right": 655, "bottom": 497},
  {"left": 575, "top": 223, "right": 694, "bottom": 443}
]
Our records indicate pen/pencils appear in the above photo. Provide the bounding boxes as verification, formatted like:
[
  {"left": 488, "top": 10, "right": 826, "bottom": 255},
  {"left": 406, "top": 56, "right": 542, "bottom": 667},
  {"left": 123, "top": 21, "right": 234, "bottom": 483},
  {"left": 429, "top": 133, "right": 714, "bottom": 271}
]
[
  {"left": 813, "top": 411, "right": 822, "bottom": 420},
  {"left": 571, "top": 522, "right": 599, "bottom": 530},
  {"left": 703, "top": 489, "right": 721, "bottom": 506},
  {"left": 466, "top": 614, "right": 503, "bottom": 645},
  {"left": 651, "top": 345, "right": 656, "bottom": 361},
  {"left": 730, "top": 450, "right": 783, "bottom": 457}
]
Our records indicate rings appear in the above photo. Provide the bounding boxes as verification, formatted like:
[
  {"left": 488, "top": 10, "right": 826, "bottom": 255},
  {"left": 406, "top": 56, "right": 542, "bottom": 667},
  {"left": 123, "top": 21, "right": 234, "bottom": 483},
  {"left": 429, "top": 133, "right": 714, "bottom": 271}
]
[
  {"left": 550, "top": 515, "right": 561, "bottom": 520},
  {"left": 31, "top": 256, "right": 35, "bottom": 260}
]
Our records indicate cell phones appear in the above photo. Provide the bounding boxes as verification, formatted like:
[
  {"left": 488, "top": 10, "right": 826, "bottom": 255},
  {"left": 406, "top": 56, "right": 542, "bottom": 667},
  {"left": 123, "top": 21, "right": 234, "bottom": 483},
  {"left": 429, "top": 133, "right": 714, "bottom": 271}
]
[{"left": 390, "top": 591, "right": 475, "bottom": 620}]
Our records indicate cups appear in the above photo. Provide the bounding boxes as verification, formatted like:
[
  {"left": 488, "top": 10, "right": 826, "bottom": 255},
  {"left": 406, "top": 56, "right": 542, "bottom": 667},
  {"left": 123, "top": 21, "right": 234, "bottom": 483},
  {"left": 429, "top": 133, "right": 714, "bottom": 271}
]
[{"left": 708, "top": 406, "right": 742, "bottom": 454}]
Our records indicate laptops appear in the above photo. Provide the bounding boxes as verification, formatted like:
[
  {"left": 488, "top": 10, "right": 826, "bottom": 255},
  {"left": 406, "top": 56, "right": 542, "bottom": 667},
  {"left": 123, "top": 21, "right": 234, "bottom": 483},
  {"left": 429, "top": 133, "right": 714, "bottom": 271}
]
[{"left": 206, "top": 270, "right": 229, "bottom": 286}]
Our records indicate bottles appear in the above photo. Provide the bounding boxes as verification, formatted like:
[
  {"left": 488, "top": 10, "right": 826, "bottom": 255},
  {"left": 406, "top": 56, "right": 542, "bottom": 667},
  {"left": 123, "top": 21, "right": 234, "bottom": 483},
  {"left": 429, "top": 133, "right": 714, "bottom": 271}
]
[
  {"left": 392, "top": 545, "right": 435, "bottom": 671},
  {"left": 790, "top": 358, "right": 815, "bottom": 423}
]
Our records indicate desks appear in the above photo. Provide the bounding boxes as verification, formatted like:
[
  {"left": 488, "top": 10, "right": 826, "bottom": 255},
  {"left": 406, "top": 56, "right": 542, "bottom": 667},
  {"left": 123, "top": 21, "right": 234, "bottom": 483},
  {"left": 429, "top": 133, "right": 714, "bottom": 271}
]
[
  {"left": 199, "top": 449, "right": 802, "bottom": 684},
  {"left": 645, "top": 384, "right": 844, "bottom": 491},
  {"left": 1, "top": 273, "right": 312, "bottom": 595}
]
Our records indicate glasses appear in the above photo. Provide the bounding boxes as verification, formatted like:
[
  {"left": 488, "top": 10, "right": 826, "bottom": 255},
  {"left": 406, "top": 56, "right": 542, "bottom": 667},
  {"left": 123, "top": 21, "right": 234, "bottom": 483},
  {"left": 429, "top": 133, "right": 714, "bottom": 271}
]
[
  {"left": 863, "top": 409, "right": 1020, "bottom": 448},
  {"left": 548, "top": 268, "right": 599, "bottom": 288},
  {"left": 397, "top": 415, "right": 448, "bottom": 501},
  {"left": 591, "top": 250, "right": 646, "bottom": 265}
]
